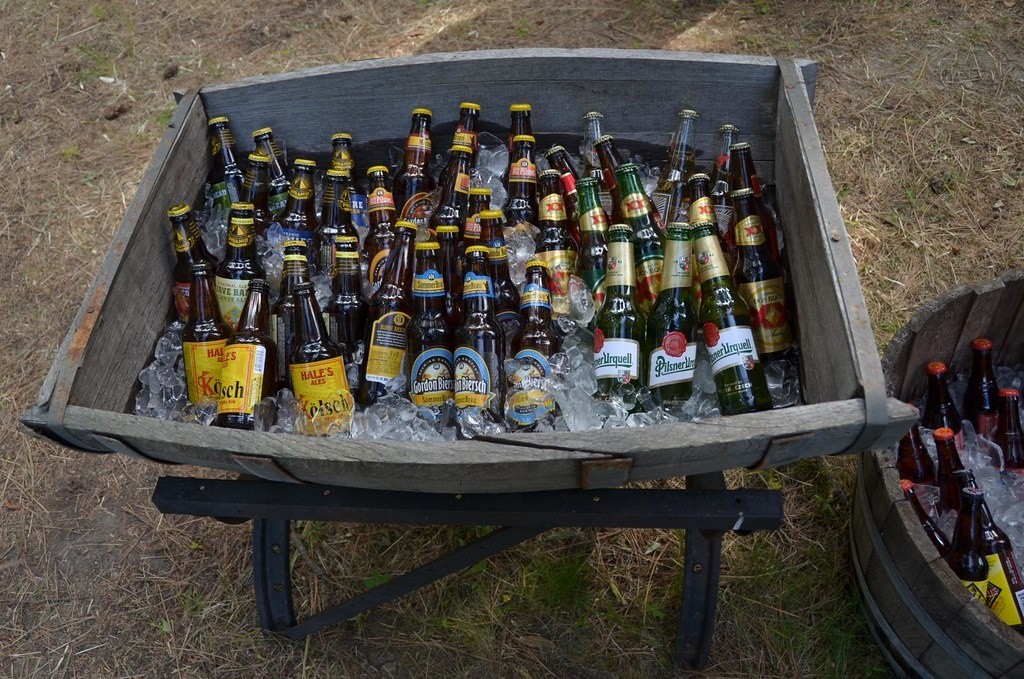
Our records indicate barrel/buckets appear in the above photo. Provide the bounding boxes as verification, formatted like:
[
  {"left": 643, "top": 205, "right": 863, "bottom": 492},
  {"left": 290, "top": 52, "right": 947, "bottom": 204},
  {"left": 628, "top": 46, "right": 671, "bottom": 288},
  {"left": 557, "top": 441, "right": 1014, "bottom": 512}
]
[{"left": 850, "top": 271, "right": 1024, "bottom": 679}]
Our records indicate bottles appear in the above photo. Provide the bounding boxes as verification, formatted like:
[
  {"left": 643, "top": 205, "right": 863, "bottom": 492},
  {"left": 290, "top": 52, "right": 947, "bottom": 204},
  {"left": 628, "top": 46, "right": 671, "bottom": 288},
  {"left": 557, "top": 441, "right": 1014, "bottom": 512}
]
[
  {"left": 896, "top": 335, "right": 1024, "bottom": 640},
  {"left": 168, "top": 101, "right": 802, "bottom": 437}
]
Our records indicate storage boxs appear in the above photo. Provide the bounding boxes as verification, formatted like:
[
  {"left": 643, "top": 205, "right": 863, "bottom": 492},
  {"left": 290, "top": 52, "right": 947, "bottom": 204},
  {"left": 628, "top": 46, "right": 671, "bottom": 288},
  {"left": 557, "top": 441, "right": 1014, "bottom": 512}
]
[{"left": 18, "top": 48, "right": 918, "bottom": 496}]
